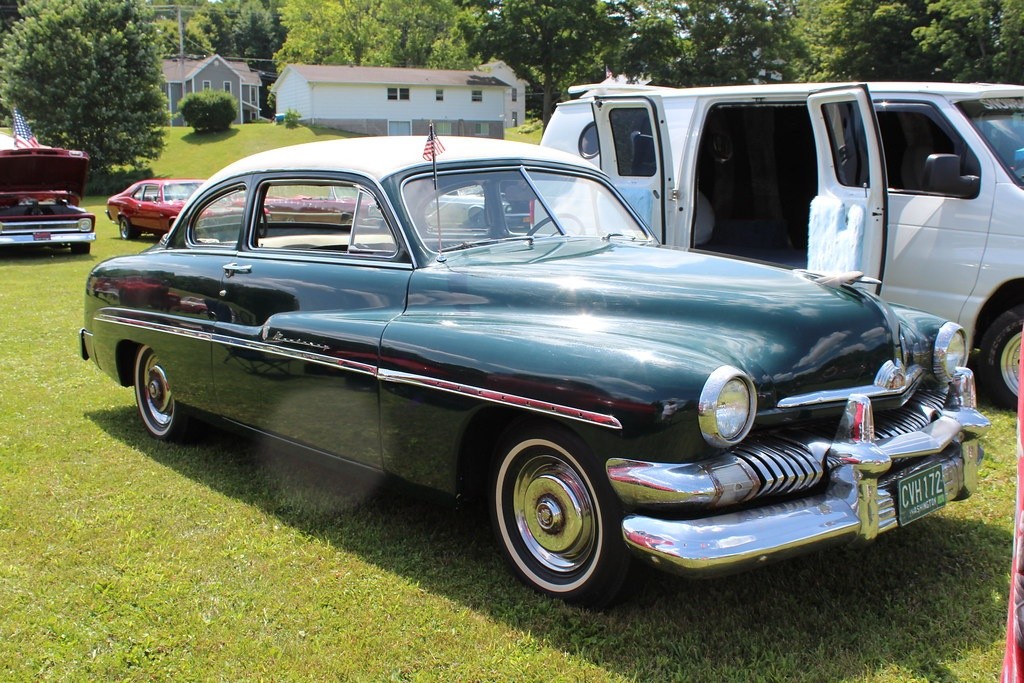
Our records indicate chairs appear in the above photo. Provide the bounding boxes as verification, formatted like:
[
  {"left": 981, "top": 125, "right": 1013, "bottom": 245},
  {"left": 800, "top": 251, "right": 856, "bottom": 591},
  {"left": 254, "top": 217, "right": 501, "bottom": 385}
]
[{"left": 901, "top": 142, "right": 935, "bottom": 190}]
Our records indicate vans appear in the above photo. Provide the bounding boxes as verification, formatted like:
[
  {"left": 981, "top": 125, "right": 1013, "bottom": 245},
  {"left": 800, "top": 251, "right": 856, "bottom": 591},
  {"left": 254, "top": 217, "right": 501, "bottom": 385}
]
[{"left": 531, "top": 80, "right": 1024, "bottom": 408}]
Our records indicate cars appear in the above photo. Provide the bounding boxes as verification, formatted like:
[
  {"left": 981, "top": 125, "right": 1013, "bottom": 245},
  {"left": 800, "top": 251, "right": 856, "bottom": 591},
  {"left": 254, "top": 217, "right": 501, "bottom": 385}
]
[
  {"left": 106, "top": 179, "right": 258, "bottom": 242},
  {"left": 0, "top": 132, "right": 97, "bottom": 255},
  {"left": 77, "top": 132, "right": 991, "bottom": 615}
]
